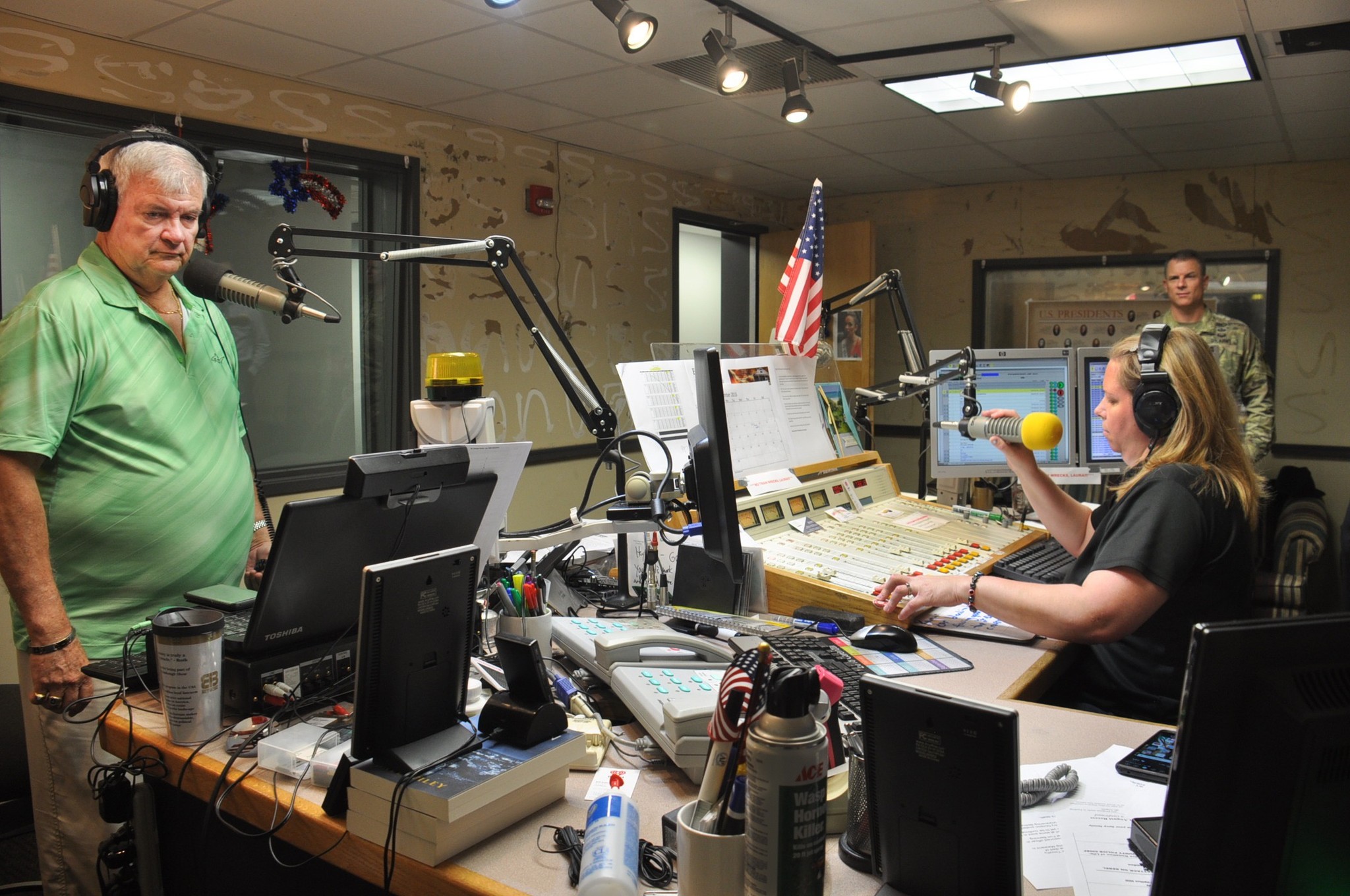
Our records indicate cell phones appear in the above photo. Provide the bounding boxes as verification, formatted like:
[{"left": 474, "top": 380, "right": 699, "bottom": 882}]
[
  {"left": 181, "top": 582, "right": 258, "bottom": 612},
  {"left": 1115, "top": 729, "right": 1177, "bottom": 784}
]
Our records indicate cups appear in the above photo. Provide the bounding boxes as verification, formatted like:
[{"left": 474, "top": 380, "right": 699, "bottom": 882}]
[
  {"left": 498, "top": 606, "right": 554, "bottom": 670},
  {"left": 676, "top": 800, "right": 746, "bottom": 896},
  {"left": 151, "top": 606, "right": 225, "bottom": 747}
]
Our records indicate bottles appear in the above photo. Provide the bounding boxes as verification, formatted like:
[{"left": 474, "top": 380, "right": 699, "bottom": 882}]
[{"left": 577, "top": 774, "right": 642, "bottom": 896}]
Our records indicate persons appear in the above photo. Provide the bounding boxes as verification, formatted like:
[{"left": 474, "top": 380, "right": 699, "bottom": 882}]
[
  {"left": 1, "top": 128, "right": 271, "bottom": 896},
  {"left": 874, "top": 324, "right": 1272, "bottom": 726},
  {"left": 1130, "top": 249, "right": 1277, "bottom": 465}
]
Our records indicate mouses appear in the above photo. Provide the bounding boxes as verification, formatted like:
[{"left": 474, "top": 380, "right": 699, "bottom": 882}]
[{"left": 847, "top": 622, "right": 917, "bottom": 652}]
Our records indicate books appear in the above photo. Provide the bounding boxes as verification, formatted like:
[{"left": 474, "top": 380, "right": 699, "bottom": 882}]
[
  {"left": 350, "top": 712, "right": 586, "bottom": 823},
  {"left": 348, "top": 780, "right": 567, "bottom": 867},
  {"left": 655, "top": 606, "right": 793, "bottom": 638},
  {"left": 348, "top": 763, "right": 570, "bottom": 843}
]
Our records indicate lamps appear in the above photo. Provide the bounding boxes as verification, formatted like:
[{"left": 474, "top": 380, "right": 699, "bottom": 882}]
[
  {"left": 778, "top": 46, "right": 817, "bottom": 123},
  {"left": 700, "top": 4, "right": 753, "bottom": 98},
  {"left": 969, "top": 41, "right": 1033, "bottom": 117},
  {"left": 1213, "top": 268, "right": 1232, "bottom": 288},
  {"left": 482, "top": 0, "right": 522, "bottom": 11},
  {"left": 588, "top": 0, "right": 661, "bottom": 56},
  {"left": 1138, "top": 279, "right": 1150, "bottom": 293}
]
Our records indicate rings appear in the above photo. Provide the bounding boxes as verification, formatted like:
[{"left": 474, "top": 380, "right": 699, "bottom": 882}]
[
  {"left": 906, "top": 583, "right": 911, "bottom": 596},
  {"left": 34, "top": 692, "right": 46, "bottom": 702},
  {"left": 48, "top": 696, "right": 63, "bottom": 707}
]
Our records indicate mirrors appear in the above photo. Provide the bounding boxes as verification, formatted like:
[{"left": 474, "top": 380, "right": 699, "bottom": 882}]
[{"left": 968, "top": 245, "right": 1283, "bottom": 436}]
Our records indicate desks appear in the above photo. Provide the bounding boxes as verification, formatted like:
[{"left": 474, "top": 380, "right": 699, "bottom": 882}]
[{"left": 95, "top": 617, "right": 1181, "bottom": 896}]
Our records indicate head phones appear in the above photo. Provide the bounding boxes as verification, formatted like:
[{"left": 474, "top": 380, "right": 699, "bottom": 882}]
[
  {"left": 1132, "top": 323, "right": 1181, "bottom": 438},
  {"left": 78, "top": 131, "right": 215, "bottom": 239}
]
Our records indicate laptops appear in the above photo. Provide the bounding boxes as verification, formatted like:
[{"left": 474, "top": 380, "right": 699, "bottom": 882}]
[{"left": 146, "top": 469, "right": 493, "bottom": 659}]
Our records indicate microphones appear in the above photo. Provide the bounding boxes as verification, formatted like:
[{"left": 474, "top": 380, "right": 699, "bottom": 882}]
[
  {"left": 182, "top": 258, "right": 341, "bottom": 323},
  {"left": 932, "top": 411, "right": 1062, "bottom": 450},
  {"left": 211, "top": 158, "right": 225, "bottom": 200}
]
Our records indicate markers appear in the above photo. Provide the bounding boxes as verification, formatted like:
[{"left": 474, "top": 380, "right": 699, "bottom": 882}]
[
  {"left": 952, "top": 505, "right": 1003, "bottom": 521},
  {"left": 776, "top": 615, "right": 839, "bottom": 635}
]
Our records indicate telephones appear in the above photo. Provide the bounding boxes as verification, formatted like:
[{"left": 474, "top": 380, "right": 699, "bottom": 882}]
[
  {"left": 552, "top": 619, "right": 736, "bottom": 687},
  {"left": 611, "top": 665, "right": 727, "bottom": 784}
]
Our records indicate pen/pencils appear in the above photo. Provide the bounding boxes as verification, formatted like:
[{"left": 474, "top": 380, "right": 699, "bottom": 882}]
[{"left": 495, "top": 550, "right": 547, "bottom": 617}]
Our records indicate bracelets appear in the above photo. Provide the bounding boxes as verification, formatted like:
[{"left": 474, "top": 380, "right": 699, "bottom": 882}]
[
  {"left": 29, "top": 626, "right": 77, "bottom": 656},
  {"left": 968, "top": 571, "right": 984, "bottom": 613},
  {"left": 253, "top": 519, "right": 267, "bottom": 533}
]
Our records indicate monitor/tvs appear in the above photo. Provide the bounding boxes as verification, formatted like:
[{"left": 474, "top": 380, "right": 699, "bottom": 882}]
[
  {"left": 858, "top": 673, "right": 1024, "bottom": 895},
  {"left": 1146, "top": 610, "right": 1350, "bottom": 895},
  {"left": 928, "top": 347, "right": 1077, "bottom": 475},
  {"left": 350, "top": 543, "right": 483, "bottom": 772},
  {"left": 1077, "top": 342, "right": 1132, "bottom": 474}
]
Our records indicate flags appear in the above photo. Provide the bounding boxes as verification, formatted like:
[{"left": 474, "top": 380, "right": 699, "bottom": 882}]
[{"left": 775, "top": 177, "right": 825, "bottom": 358}]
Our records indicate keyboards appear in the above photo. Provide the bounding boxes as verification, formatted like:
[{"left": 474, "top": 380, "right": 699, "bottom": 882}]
[
  {"left": 991, "top": 535, "right": 1078, "bottom": 585},
  {"left": 79, "top": 648, "right": 150, "bottom": 691}
]
